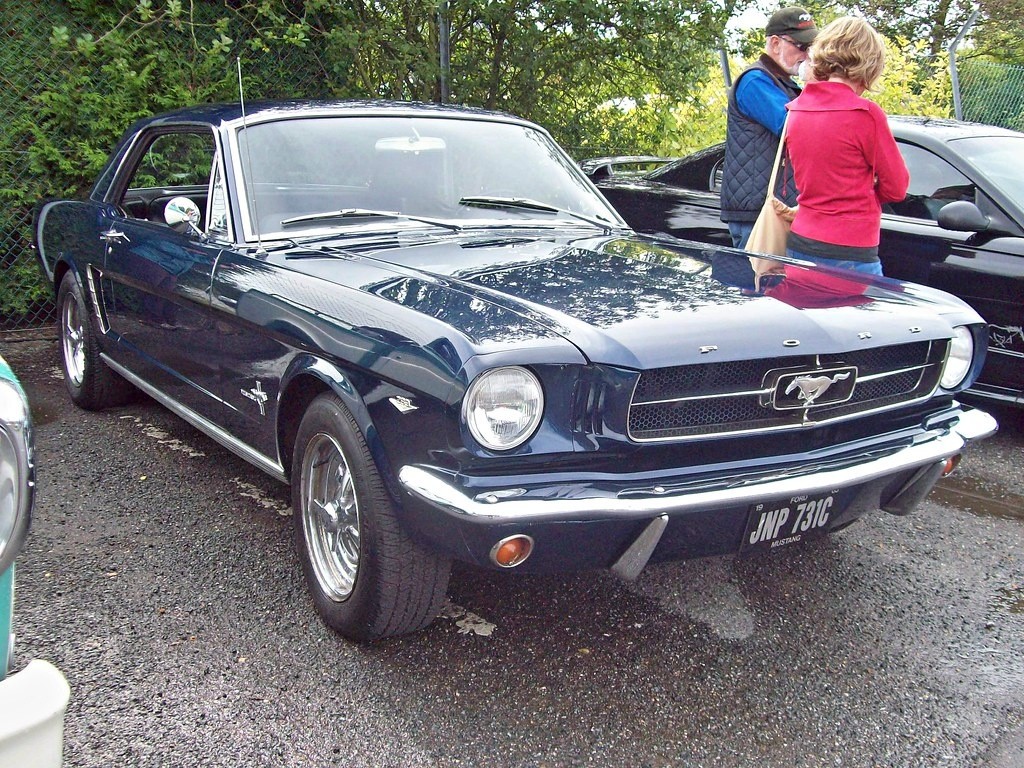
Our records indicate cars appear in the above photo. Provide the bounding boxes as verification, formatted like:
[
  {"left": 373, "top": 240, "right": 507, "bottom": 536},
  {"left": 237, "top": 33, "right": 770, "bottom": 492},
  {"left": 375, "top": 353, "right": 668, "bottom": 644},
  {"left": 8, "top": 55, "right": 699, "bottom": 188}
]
[
  {"left": 1, "top": 357, "right": 70, "bottom": 763},
  {"left": 34, "top": 99, "right": 998, "bottom": 642},
  {"left": 578, "top": 115, "right": 1024, "bottom": 414}
]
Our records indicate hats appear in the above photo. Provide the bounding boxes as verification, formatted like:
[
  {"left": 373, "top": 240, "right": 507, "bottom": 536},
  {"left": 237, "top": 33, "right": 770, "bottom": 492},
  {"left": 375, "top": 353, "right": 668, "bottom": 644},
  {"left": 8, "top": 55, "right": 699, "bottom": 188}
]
[{"left": 767, "top": 8, "right": 820, "bottom": 43}]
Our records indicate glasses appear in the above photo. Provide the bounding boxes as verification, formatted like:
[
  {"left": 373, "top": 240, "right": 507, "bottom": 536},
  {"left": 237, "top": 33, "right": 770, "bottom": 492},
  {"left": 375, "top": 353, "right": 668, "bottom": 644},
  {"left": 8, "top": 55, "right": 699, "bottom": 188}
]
[{"left": 779, "top": 35, "right": 811, "bottom": 51}]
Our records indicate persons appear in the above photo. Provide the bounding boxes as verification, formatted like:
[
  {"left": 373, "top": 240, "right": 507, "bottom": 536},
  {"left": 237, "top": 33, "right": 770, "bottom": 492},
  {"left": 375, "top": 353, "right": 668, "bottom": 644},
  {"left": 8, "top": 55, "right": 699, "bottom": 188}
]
[
  {"left": 721, "top": 6, "right": 820, "bottom": 250},
  {"left": 765, "top": 265, "right": 875, "bottom": 310},
  {"left": 786, "top": 15, "right": 909, "bottom": 275},
  {"left": 710, "top": 251, "right": 785, "bottom": 297}
]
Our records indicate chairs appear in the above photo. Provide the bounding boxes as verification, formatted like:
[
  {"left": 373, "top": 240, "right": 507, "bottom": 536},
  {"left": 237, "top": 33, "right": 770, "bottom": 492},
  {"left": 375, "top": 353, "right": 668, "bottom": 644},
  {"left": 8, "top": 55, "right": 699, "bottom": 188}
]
[{"left": 364, "top": 144, "right": 450, "bottom": 219}]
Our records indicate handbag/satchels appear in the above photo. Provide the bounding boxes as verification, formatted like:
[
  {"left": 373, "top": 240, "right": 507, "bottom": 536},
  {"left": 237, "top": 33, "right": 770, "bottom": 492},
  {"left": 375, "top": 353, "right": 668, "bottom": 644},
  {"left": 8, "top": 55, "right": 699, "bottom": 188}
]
[{"left": 745, "top": 193, "right": 799, "bottom": 292}]
